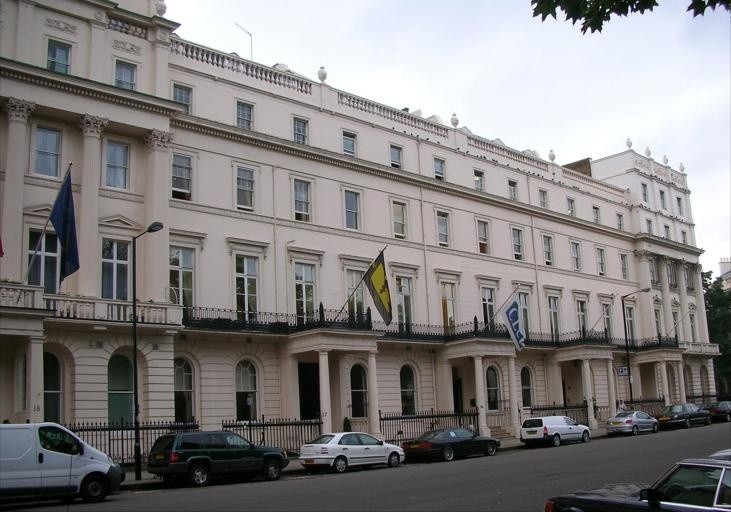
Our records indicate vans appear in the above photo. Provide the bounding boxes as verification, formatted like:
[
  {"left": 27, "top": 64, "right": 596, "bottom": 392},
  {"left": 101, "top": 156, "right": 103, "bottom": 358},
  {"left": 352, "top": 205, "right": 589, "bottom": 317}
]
[{"left": 0, "top": 422, "right": 124, "bottom": 501}]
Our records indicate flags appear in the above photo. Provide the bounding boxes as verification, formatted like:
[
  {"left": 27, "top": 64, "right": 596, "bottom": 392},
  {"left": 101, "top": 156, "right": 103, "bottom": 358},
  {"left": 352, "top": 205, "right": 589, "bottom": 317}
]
[
  {"left": 48, "top": 170, "right": 80, "bottom": 288},
  {"left": 499, "top": 292, "right": 526, "bottom": 353},
  {"left": 362, "top": 252, "right": 392, "bottom": 326}
]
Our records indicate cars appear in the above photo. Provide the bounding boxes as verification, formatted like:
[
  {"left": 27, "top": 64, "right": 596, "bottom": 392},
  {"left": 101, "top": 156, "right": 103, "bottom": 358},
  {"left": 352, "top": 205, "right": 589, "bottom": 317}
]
[
  {"left": 606, "top": 401, "right": 731, "bottom": 436},
  {"left": 520, "top": 416, "right": 591, "bottom": 447},
  {"left": 298, "top": 427, "right": 499, "bottom": 473},
  {"left": 546, "top": 449, "right": 731, "bottom": 512},
  {"left": 147, "top": 431, "right": 289, "bottom": 486}
]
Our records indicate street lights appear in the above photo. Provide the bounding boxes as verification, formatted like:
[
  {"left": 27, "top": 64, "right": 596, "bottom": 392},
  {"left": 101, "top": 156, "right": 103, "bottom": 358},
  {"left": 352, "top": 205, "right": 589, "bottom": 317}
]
[
  {"left": 620, "top": 288, "right": 652, "bottom": 404},
  {"left": 132, "top": 222, "right": 163, "bottom": 481}
]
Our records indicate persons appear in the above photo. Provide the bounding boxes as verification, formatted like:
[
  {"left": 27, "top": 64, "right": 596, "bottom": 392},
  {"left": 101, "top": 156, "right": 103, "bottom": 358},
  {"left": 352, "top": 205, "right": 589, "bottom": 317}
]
[{"left": 618, "top": 399, "right": 627, "bottom": 414}]
